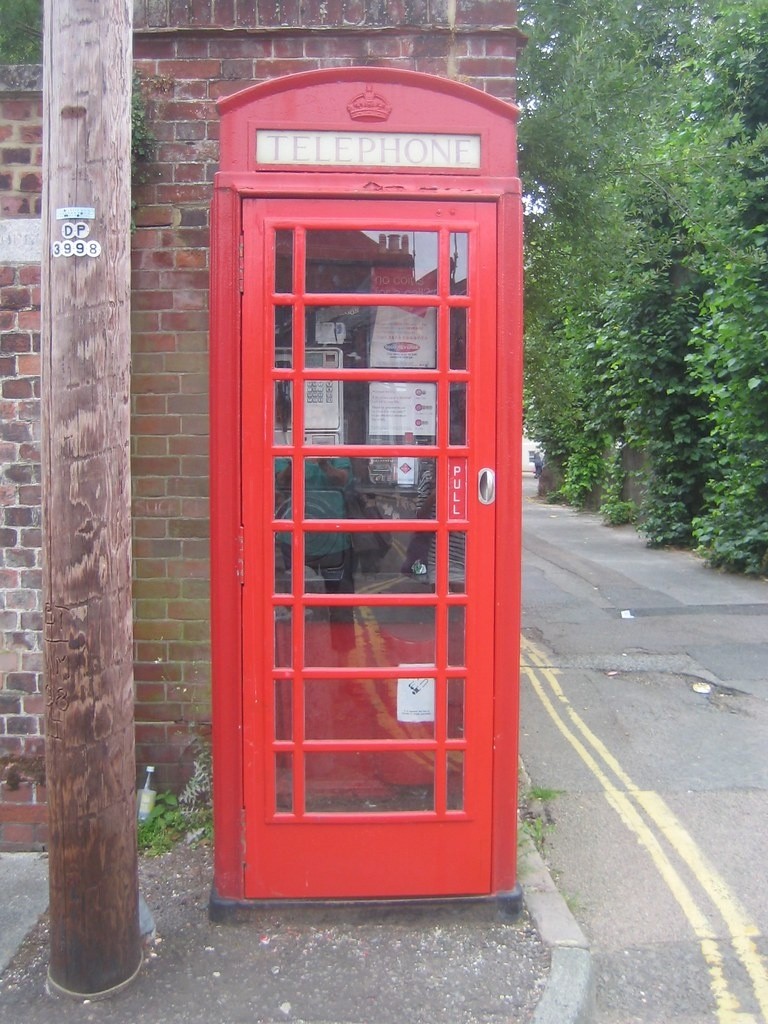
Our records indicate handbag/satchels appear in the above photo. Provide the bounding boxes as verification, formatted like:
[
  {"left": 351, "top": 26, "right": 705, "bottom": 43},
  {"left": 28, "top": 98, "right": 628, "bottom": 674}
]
[
  {"left": 401, "top": 535, "right": 431, "bottom": 586},
  {"left": 360, "top": 504, "right": 393, "bottom": 561}
]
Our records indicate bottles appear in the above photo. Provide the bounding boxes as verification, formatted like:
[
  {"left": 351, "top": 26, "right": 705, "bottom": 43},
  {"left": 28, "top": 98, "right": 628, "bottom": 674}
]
[{"left": 137, "top": 764, "right": 157, "bottom": 823}]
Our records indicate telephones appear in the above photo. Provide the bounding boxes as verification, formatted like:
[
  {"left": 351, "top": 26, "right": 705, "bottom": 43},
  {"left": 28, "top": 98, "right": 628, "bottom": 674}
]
[{"left": 273, "top": 348, "right": 345, "bottom": 458}]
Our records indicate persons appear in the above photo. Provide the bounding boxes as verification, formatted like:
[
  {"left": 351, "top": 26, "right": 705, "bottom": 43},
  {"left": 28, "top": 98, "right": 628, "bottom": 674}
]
[
  {"left": 401, "top": 459, "right": 435, "bottom": 586},
  {"left": 275, "top": 456, "right": 355, "bottom": 667},
  {"left": 428, "top": 531, "right": 465, "bottom": 596}
]
